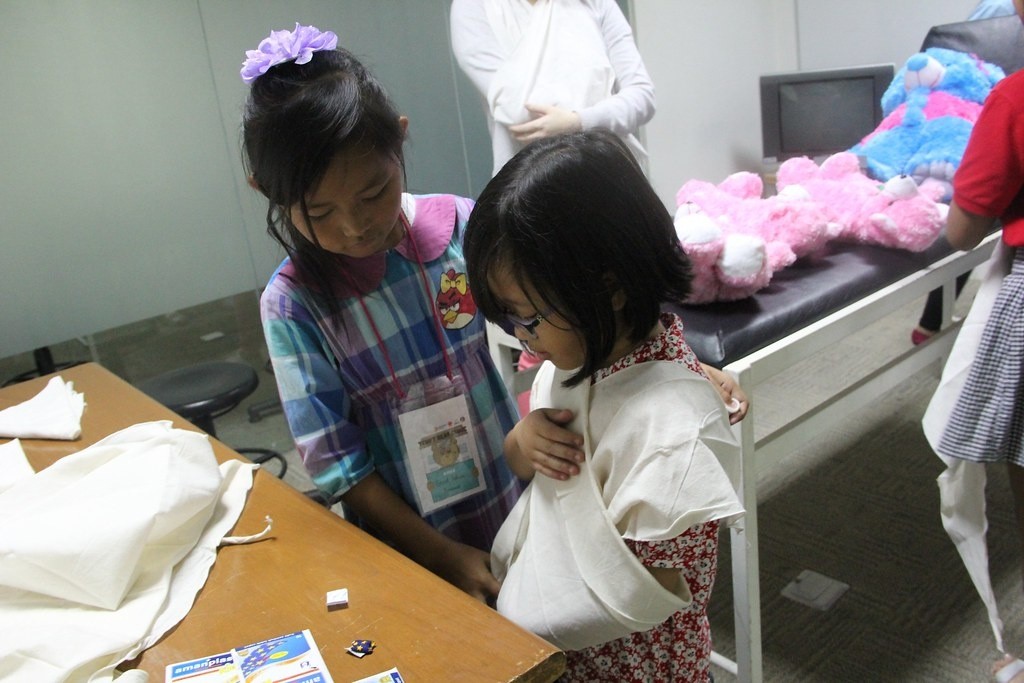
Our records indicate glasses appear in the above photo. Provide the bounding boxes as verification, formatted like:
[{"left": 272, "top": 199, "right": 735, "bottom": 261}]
[{"left": 505, "top": 300, "right": 562, "bottom": 339}]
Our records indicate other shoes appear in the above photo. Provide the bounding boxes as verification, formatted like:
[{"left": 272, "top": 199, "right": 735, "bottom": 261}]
[{"left": 911, "top": 329, "right": 931, "bottom": 345}]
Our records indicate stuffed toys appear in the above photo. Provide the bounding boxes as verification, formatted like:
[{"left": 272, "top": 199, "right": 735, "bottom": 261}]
[{"left": 671, "top": 47, "right": 1008, "bottom": 306}]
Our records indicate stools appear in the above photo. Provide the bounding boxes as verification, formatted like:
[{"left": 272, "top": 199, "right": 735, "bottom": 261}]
[{"left": 149, "top": 362, "right": 286, "bottom": 480}]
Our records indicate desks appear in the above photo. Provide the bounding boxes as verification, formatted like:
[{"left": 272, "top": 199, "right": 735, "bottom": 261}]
[
  {"left": 661, "top": 231, "right": 1000, "bottom": 683},
  {"left": 0, "top": 361, "right": 570, "bottom": 683}
]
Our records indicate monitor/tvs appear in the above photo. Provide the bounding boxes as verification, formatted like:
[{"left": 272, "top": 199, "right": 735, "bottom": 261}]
[{"left": 760, "top": 64, "right": 894, "bottom": 174}]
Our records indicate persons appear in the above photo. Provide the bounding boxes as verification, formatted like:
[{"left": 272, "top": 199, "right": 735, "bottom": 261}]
[
  {"left": 460, "top": 129, "right": 744, "bottom": 682},
  {"left": 238, "top": 48, "right": 751, "bottom": 609},
  {"left": 909, "top": 2, "right": 1024, "bottom": 682},
  {"left": 447, "top": 0, "right": 661, "bottom": 179}
]
